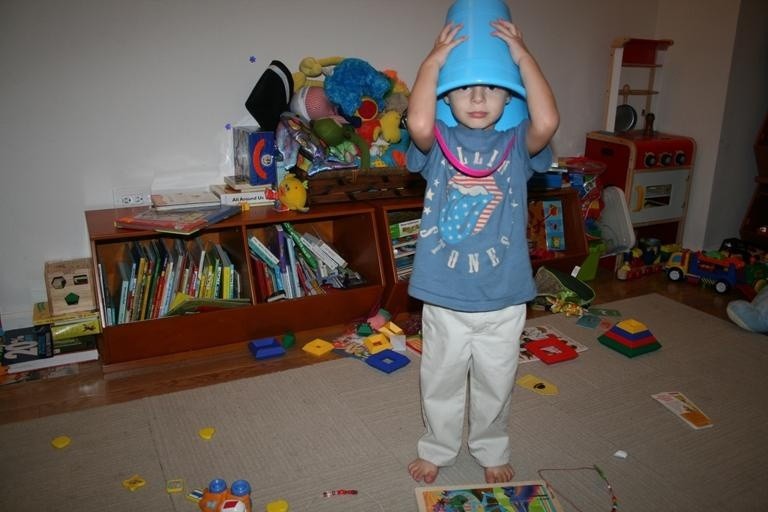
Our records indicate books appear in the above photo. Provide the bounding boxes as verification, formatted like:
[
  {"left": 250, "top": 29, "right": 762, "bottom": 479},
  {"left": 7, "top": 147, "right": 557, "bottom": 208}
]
[
  {"left": 98, "top": 224, "right": 364, "bottom": 327},
  {"left": 2, "top": 325, "right": 99, "bottom": 373},
  {"left": 115, "top": 175, "right": 274, "bottom": 236},
  {"left": 415, "top": 479, "right": 560, "bottom": 512}
]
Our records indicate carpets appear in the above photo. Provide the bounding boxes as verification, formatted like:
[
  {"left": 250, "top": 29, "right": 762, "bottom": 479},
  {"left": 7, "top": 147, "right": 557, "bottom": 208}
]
[{"left": 0, "top": 292, "right": 768, "bottom": 512}]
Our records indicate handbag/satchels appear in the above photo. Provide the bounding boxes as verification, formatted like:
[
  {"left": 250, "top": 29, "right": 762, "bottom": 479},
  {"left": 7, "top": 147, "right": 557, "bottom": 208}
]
[{"left": 535, "top": 259, "right": 595, "bottom": 312}]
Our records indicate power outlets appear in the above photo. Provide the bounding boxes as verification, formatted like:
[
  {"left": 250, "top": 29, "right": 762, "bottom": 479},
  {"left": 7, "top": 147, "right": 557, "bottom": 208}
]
[{"left": 113, "top": 185, "right": 152, "bottom": 207}]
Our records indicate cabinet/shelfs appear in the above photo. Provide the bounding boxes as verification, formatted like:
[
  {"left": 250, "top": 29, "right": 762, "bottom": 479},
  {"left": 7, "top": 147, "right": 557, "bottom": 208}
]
[
  {"left": 241, "top": 200, "right": 388, "bottom": 342},
  {"left": 365, "top": 196, "right": 431, "bottom": 322},
  {"left": 527, "top": 188, "right": 590, "bottom": 278},
  {"left": 84, "top": 205, "right": 257, "bottom": 366}
]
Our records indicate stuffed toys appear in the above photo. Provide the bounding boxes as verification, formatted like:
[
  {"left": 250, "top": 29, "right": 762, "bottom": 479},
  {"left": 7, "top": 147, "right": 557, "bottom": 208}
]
[
  {"left": 727, "top": 289, "right": 768, "bottom": 333},
  {"left": 289, "top": 54, "right": 411, "bottom": 173}
]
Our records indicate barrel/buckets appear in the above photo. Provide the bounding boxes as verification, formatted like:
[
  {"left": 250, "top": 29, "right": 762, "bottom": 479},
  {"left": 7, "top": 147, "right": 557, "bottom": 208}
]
[{"left": 436, "top": 0, "right": 530, "bottom": 178}]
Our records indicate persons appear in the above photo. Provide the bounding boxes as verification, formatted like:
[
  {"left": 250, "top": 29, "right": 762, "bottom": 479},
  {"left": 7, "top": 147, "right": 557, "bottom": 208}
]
[{"left": 407, "top": 19, "right": 559, "bottom": 484}]
